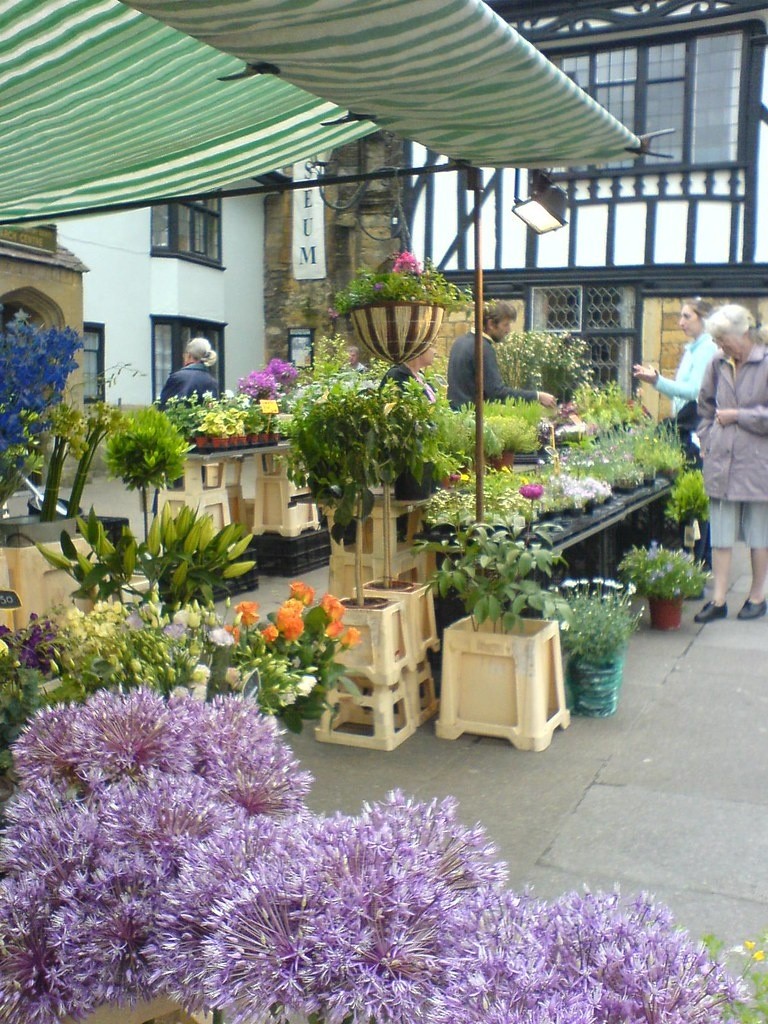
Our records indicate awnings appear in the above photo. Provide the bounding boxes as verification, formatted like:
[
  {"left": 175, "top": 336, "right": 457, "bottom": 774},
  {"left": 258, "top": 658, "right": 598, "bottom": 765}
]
[{"left": 0, "top": 0, "right": 642, "bottom": 228}]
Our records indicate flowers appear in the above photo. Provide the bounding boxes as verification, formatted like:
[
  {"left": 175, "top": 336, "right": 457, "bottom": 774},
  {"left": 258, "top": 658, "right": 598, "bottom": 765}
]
[
  {"left": 152, "top": 390, "right": 265, "bottom": 436},
  {"left": 0, "top": 576, "right": 768, "bottom": 1024},
  {"left": 405, "top": 474, "right": 573, "bottom": 631},
  {"left": 615, "top": 541, "right": 713, "bottom": 601},
  {"left": 555, "top": 577, "right": 646, "bottom": 666},
  {"left": 328, "top": 250, "right": 475, "bottom": 318},
  {"left": 602, "top": 417, "right": 691, "bottom": 486},
  {"left": 493, "top": 329, "right": 645, "bottom": 426}
]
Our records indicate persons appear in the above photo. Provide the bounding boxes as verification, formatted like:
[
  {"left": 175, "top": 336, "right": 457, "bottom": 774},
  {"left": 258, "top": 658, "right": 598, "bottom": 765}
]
[
  {"left": 157, "top": 338, "right": 220, "bottom": 488},
  {"left": 380, "top": 345, "right": 437, "bottom": 530},
  {"left": 342, "top": 347, "right": 366, "bottom": 371},
  {"left": 693, "top": 306, "right": 768, "bottom": 623},
  {"left": 632, "top": 299, "right": 721, "bottom": 602},
  {"left": 447, "top": 301, "right": 557, "bottom": 414}
]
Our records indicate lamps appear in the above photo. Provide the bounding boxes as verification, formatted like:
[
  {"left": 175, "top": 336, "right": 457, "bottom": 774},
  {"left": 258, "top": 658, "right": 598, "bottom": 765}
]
[{"left": 511, "top": 168, "right": 570, "bottom": 234}]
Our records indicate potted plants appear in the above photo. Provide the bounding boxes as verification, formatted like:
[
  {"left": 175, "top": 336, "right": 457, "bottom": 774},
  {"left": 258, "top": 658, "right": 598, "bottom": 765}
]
[
  {"left": 281, "top": 367, "right": 538, "bottom": 752},
  {"left": 0, "top": 363, "right": 158, "bottom": 632},
  {"left": 617, "top": 479, "right": 656, "bottom": 494}
]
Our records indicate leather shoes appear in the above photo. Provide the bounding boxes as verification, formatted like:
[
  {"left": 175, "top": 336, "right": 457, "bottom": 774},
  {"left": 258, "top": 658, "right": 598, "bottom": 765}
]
[
  {"left": 737, "top": 598, "right": 767, "bottom": 619},
  {"left": 694, "top": 599, "right": 727, "bottom": 623}
]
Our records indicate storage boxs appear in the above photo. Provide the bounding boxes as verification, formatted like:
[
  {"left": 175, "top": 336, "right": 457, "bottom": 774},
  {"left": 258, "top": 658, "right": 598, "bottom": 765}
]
[
  {"left": 250, "top": 527, "right": 332, "bottom": 578},
  {"left": 160, "top": 545, "right": 260, "bottom": 608}
]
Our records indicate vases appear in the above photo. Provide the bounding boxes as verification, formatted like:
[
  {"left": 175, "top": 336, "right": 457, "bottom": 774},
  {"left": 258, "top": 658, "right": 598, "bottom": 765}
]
[
  {"left": 193, "top": 433, "right": 279, "bottom": 446},
  {"left": 649, "top": 595, "right": 685, "bottom": 631},
  {"left": 350, "top": 302, "right": 447, "bottom": 367},
  {"left": 433, "top": 613, "right": 573, "bottom": 751},
  {"left": 568, "top": 641, "right": 626, "bottom": 719}
]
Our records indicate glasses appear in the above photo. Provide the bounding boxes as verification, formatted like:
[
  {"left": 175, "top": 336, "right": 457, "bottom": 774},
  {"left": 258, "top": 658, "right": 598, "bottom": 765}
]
[{"left": 712, "top": 332, "right": 737, "bottom": 345}]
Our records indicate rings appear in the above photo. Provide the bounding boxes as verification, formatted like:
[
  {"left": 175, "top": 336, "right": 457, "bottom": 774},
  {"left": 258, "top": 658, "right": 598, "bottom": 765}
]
[{"left": 717, "top": 418, "right": 719, "bottom": 422}]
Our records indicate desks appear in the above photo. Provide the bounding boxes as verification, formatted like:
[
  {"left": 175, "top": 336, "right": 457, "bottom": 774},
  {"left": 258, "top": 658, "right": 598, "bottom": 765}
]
[{"left": 412, "top": 477, "right": 671, "bottom": 576}]
